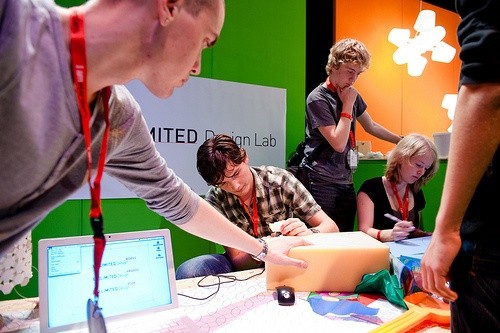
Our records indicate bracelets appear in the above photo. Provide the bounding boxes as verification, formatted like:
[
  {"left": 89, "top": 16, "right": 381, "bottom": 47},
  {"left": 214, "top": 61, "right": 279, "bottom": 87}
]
[
  {"left": 377, "top": 230, "right": 382, "bottom": 241},
  {"left": 251, "top": 238, "right": 269, "bottom": 262},
  {"left": 310, "top": 228, "right": 321, "bottom": 233},
  {"left": 341, "top": 112, "right": 353, "bottom": 120}
]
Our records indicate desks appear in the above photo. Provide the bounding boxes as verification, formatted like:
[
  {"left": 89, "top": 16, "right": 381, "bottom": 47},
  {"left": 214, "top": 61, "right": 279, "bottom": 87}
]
[
  {"left": 0, "top": 234, "right": 452, "bottom": 333},
  {"left": 352, "top": 158, "right": 448, "bottom": 233}
]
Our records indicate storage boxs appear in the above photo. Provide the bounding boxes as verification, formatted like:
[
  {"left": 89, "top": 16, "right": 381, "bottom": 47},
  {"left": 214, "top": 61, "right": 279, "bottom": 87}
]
[
  {"left": 400, "top": 252, "right": 424, "bottom": 288},
  {"left": 265, "top": 231, "right": 390, "bottom": 293}
]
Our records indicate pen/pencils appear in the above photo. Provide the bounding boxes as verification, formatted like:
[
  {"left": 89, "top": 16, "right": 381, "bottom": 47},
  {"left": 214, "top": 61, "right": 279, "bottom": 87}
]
[{"left": 384, "top": 213, "right": 416, "bottom": 230}]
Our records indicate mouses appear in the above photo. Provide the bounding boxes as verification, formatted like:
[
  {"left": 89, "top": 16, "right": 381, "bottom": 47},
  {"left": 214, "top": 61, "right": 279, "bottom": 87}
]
[{"left": 275, "top": 285, "right": 295, "bottom": 306}]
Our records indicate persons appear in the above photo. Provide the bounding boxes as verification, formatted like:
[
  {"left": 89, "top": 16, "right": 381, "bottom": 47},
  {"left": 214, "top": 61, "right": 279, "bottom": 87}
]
[
  {"left": 357, "top": 134, "right": 440, "bottom": 243},
  {"left": 0, "top": 0, "right": 313, "bottom": 269},
  {"left": 419, "top": 0, "right": 500, "bottom": 333},
  {"left": 175, "top": 135, "right": 340, "bottom": 280},
  {"left": 303, "top": 38, "right": 405, "bottom": 232}
]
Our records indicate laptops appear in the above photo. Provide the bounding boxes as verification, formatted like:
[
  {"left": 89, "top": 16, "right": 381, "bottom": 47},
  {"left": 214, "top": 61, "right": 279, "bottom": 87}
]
[{"left": 38, "top": 228, "right": 204, "bottom": 333}]
